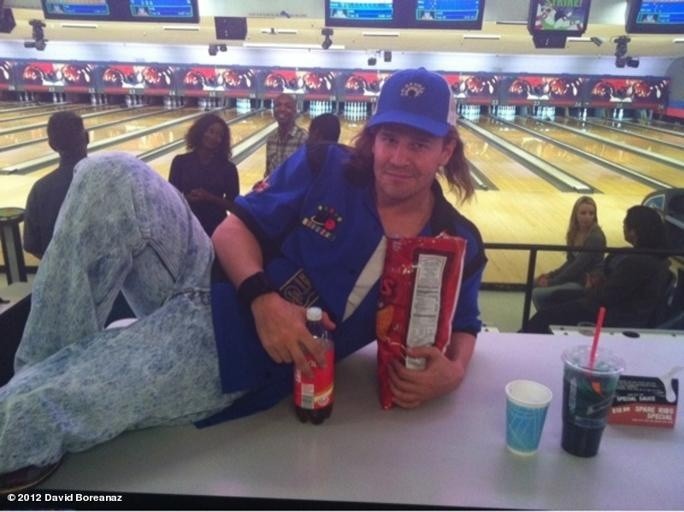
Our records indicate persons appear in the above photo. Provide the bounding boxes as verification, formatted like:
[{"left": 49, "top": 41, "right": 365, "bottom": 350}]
[
  {"left": 310, "top": 115, "right": 341, "bottom": 144},
  {"left": 262, "top": 92, "right": 308, "bottom": 180},
  {"left": 514, "top": 204, "right": 672, "bottom": 336},
  {"left": 532, "top": 195, "right": 607, "bottom": 312},
  {"left": 168, "top": 115, "right": 238, "bottom": 238},
  {"left": 0, "top": 65, "right": 487, "bottom": 475},
  {"left": 22, "top": 111, "right": 89, "bottom": 259}
]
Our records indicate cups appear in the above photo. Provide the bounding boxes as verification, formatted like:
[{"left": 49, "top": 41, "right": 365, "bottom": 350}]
[
  {"left": 503, "top": 379, "right": 553, "bottom": 457},
  {"left": 559, "top": 345, "right": 627, "bottom": 455}
]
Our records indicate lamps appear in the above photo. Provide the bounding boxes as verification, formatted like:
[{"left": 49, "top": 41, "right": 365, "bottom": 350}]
[
  {"left": 366, "top": 48, "right": 393, "bottom": 66},
  {"left": 613, "top": 35, "right": 639, "bottom": 69},
  {"left": 320, "top": 29, "right": 334, "bottom": 50},
  {"left": 591, "top": 37, "right": 605, "bottom": 49},
  {"left": 208, "top": 43, "right": 227, "bottom": 57}
]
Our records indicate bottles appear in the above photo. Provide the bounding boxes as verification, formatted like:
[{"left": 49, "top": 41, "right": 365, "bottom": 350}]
[{"left": 292, "top": 306, "right": 334, "bottom": 425}]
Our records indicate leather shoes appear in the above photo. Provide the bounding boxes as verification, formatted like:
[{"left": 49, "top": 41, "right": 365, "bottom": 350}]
[{"left": 0, "top": 457, "right": 61, "bottom": 496}]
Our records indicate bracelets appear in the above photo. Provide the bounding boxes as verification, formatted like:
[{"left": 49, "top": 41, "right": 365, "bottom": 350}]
[{"left": 234, "top": 270, "right": 275, "bottom": 310}]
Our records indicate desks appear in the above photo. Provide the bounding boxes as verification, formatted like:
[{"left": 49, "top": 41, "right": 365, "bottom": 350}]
[{"left": 36, "top": 315, "right": 684, "bottom": 512}]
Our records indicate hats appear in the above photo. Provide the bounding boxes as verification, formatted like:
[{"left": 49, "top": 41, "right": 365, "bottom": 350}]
[{"left": 363, "top": 65, "right": 458, "bottom": 139}]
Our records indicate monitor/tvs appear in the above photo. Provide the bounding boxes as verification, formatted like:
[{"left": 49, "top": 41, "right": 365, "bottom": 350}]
[
  {"left": 527, "top": 0, "right": 590, "bottom": 35},
  {"left": 127, "top": 0, "right": 198, "bottom": 22},
  {"left": 625, "top": 0, "right": 684, "bottom": 34},
  {"left": 324, "top": 0, "right": 404, "bottom": 28},
  {"left": 404, "top": 0, "right": 484, "bottom": 30},
  {"left": 41, "top": 0, "right": 112, "bottom": 20}
]
